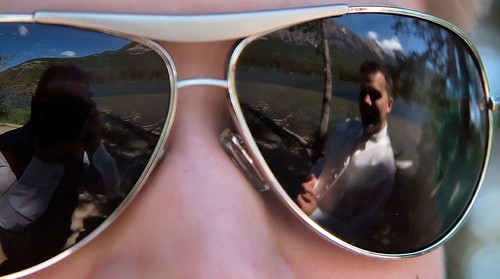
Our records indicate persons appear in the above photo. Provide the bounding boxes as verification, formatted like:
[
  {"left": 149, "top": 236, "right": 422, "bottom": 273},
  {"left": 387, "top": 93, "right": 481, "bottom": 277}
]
[
  {"left": 1, "top": 0, "right": 479, "bottom": 279},
  {"left": 1, "top": 64, "right": 122, "bottom": 270},
  {"left": 297, "top": 59, "right": 398, "bottom": 241}
]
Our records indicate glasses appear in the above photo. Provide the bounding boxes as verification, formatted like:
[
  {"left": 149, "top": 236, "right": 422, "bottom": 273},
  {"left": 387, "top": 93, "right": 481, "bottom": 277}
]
[{"left": 0, "top": 5, "right": 499, "bottom": 279}]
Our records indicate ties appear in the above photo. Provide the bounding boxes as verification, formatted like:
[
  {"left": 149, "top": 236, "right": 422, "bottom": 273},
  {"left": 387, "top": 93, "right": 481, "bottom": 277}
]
[{"left": 314, "top": 136, "right": 368, "bottom": 202}]
